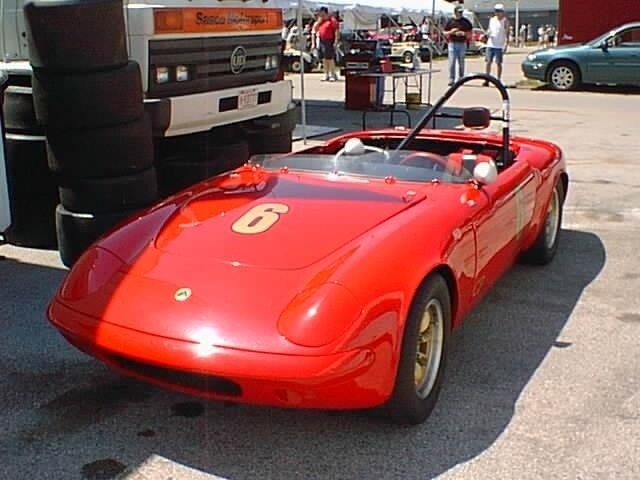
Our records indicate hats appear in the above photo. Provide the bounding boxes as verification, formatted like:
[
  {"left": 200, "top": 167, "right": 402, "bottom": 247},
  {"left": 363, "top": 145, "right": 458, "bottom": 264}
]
[
  {"left": 493, "top": 3, "right": 504, "bottom": 11},
  {"left": 454, "top": 5, "right": 464, "bottom": 12}
]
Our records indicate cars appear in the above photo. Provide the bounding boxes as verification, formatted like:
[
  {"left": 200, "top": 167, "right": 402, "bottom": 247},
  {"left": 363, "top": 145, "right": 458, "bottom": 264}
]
[{"left": 520, "top": 21, "right": 639, "bottom": 92}]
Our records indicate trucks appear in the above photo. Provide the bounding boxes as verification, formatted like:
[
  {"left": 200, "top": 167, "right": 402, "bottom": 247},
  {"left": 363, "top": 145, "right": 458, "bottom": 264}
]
[{"left": 1, "top": 1, "right": 294, "bottom": 146}]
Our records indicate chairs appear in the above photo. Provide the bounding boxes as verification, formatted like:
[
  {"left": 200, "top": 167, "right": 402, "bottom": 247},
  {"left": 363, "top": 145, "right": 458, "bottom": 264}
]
[{"left": 337, "top": 142, "right": 495, "bottom": 183}]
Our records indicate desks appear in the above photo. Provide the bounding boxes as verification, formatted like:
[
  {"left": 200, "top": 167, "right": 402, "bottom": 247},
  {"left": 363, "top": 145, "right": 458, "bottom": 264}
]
[{"left": 364, "top": 66, "right": 440, "bottom": 109}]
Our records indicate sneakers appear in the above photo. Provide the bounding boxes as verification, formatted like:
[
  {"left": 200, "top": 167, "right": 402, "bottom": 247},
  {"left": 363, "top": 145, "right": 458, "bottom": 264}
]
[
  {"left": 319, "top": 74, "right": 338, "bottom": 82},
  {"left": 447, "top": 78, "right": 491, "bottom": 88}
]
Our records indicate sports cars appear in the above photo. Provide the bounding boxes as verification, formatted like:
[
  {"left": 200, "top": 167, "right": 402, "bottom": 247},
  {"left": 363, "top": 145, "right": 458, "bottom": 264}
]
[{"left": 44, "top": 126, "right": 570, "bottom": 425}]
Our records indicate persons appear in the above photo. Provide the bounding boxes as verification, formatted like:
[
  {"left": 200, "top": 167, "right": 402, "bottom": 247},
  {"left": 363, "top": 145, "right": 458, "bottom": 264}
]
[
  {"left": 478, "top": 3, "right": 511, "bottom": 90},
  {"left": 519, "top": 23, "right": 528, "bottom": 48},
  {"left": 314, "top": 5, "right": 339, "bottom": 83},
  {"left": 537, "top": 23, "right": 559, "bottom": 47},
  {"left": 443, "top": 6, "right": 473, "bottom": 86}
]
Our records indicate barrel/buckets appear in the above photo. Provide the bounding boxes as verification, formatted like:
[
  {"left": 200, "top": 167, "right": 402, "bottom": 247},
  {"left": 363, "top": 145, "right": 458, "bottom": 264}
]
[{"left": 407, "top": 92, "right": 420, "bottom": 109}]
